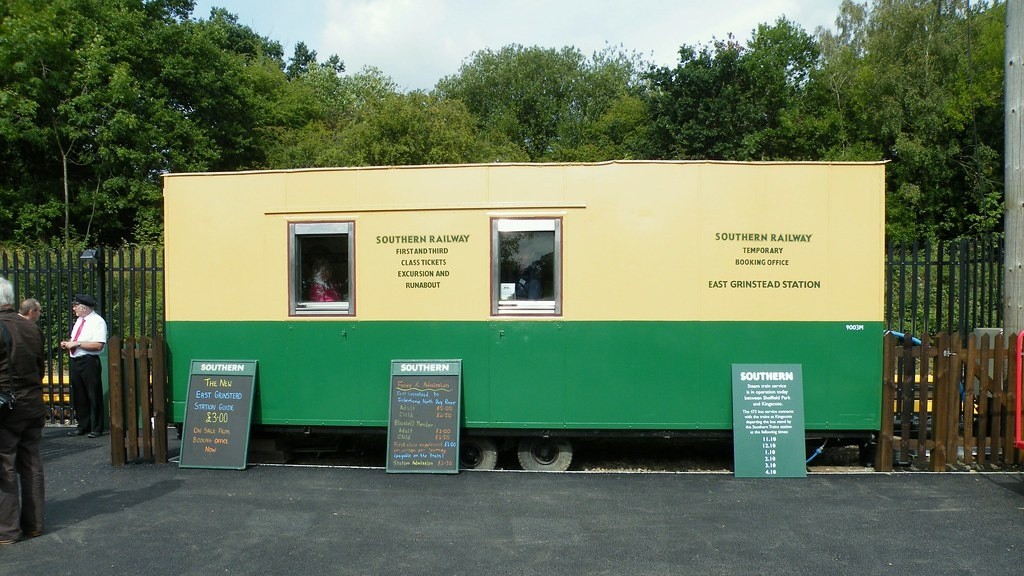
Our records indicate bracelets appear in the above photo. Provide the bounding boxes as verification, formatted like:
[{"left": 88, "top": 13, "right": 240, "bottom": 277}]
[{"left": 78, "top": 341, "right": 81, "bottom": 347}]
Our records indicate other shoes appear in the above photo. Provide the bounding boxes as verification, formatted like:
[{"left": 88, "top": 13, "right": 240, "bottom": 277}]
[
  {"left": 68, "top": 430, "right": 86, "bottom": 436},
  {"left": 23, "top": 529, "right": 43, "bottom": 536},
  {"left": 0, "top": 539, "right": 17, "bottom": 544},
  {"left": 87, "top": 432, "right": 103, "bottom": 437}
]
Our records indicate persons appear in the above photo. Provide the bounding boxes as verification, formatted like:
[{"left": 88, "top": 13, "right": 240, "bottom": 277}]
[
  {"left": 307, "top": 249, "right": 343, "bottom": 303},
  {"left": 1, "top": 278, "right": 49, "bottom": 543},
  {"left": 510, "top": 241, "right": 545, "bottom": 301},
  {"left": 16, "top": 298, "right": 43, "bottom": 326},
  {"left": 60, "top": 294, "right": 108, "bottom": 438}
]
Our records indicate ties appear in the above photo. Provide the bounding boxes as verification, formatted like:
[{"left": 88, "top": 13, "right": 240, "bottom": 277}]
[{"left": 71, "top": 319, "right": 86, "bottom": 354}]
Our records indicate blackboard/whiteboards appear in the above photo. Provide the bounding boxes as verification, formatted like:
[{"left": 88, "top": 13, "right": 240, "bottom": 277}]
[
  {"left": 180, "top": 358, "right": 258, "bottom": 470},
  {"left": 388, "top": 359, "right": 463, "bottom": 475}
]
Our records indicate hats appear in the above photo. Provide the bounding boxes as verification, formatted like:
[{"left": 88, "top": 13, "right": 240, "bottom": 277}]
[{"left": 73, "top": 293, "right": 97, "bottom": 307}]
